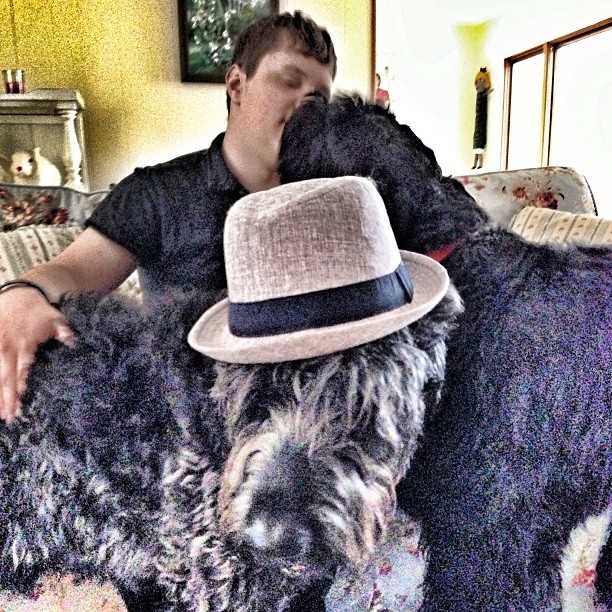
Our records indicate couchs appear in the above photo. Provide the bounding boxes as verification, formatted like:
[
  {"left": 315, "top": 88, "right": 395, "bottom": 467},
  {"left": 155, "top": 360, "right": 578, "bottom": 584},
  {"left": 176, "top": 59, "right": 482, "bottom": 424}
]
[{"left": 0, "top": 169, "right": 598, "bottom": 307}]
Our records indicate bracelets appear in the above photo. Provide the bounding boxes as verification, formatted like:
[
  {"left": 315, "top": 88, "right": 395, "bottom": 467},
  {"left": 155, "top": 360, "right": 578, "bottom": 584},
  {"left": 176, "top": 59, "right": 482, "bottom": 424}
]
[{"left": 2, "top": 274, "right": 55, "bottom": 307}]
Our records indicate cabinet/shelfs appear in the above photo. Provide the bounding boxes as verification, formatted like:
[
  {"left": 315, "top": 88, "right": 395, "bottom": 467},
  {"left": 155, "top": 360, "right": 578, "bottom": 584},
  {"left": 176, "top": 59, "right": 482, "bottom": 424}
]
[{"left": 0, "top": 87, "right": 91, "bottom": 192}]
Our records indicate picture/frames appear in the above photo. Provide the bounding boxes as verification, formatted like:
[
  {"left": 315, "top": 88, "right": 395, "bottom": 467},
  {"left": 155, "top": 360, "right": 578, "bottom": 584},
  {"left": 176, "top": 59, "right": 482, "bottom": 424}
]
[{"left": 179, "top": 0, "right": 280, "bottom": 84}]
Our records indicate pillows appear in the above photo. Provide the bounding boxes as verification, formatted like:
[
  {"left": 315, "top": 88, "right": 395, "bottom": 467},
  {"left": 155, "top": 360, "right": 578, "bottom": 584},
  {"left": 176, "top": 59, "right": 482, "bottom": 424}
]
[
  {"left": 0, "top": 219, "right": 141, "bottom": 307},
  {"left": 510, "top": 202, "right": 612, "bottom": 252}
]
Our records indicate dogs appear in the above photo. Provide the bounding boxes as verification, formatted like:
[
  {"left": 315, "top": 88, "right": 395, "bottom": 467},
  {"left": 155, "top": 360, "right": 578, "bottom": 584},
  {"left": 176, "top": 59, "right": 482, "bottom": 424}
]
[
  {"left": 0, "top": 287, "right": 465, "bottom": 612},
  {"left": 279, "top": 92, "right": 610, "bottom": 612}
]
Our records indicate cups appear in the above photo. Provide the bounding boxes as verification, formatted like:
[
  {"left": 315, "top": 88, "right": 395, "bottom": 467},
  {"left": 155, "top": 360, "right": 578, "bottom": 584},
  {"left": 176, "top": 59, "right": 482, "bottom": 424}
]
[{"left": 1, "top": 68, "right": 25, "bottom": 94}]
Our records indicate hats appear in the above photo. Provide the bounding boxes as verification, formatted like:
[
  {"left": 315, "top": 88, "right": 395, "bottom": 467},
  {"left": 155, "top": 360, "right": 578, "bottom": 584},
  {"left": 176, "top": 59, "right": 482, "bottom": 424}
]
[{"left": 187, "top": 176, "right": 449, "bottom": 365}]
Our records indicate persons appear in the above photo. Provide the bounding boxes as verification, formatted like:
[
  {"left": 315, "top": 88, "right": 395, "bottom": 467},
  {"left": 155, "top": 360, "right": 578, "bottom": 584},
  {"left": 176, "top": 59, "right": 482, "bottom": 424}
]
[{"left": 0, "top": 11, "right": 338, "bottom": 428}]
